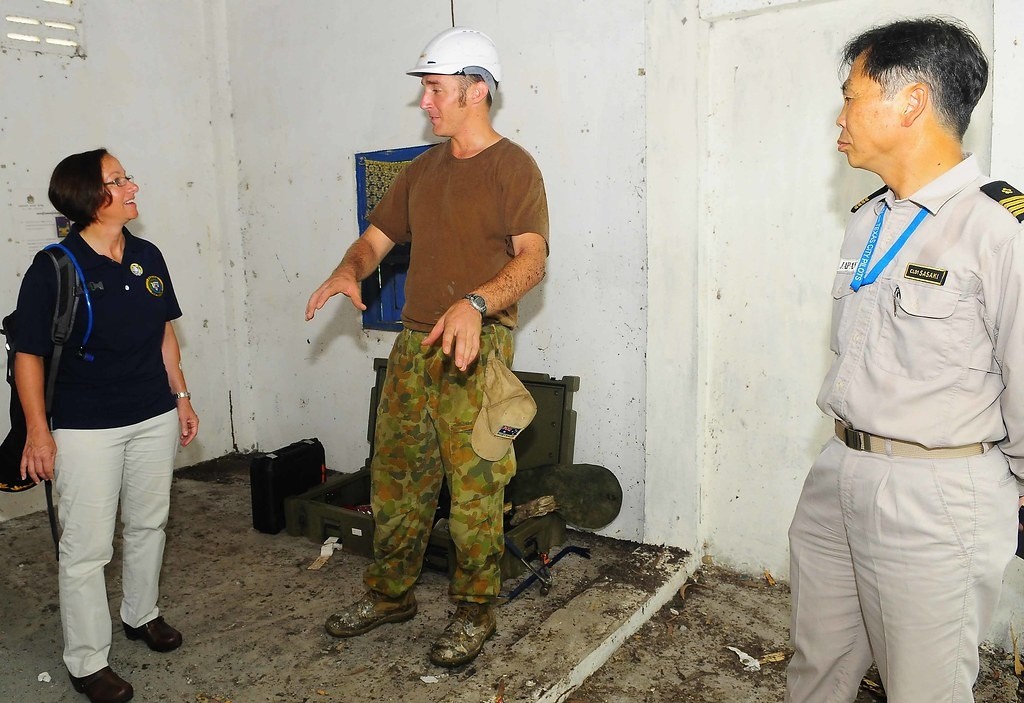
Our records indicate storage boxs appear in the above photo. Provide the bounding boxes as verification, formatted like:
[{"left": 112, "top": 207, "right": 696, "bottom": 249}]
[{"left": 251, "top": 357, "right": 580, "bottom": 586}]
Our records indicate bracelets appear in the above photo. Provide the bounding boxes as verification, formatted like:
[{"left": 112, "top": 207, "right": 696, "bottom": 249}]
[{"left": 174, "top": 392, "right": 191, "bottom": 399}]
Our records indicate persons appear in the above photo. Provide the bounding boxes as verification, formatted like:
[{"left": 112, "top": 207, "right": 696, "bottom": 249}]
[
  {"left": 304, "top": 24, "right": 549, "bottom": 668},
  {"left": 785, "top": 14, "right": 1024, "bottom": 703},
  {"left": 14, "top": 147, "right": 200, "bottom": 702}
]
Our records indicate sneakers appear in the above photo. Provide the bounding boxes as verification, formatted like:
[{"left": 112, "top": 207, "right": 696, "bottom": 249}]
[
  {"left": 324, "top": 585, "right": 418, "bottom": 637},
  {"left": 430, "top": 603, "right": 496, "bottom": 666}
]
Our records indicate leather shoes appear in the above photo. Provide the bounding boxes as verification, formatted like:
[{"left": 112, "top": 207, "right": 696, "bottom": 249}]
[
  {"left": 122, "top": 616, "right": 182, "bottom": 651},
  {"left": 67, "top": 666, "right": 133, "bottom": 703}
]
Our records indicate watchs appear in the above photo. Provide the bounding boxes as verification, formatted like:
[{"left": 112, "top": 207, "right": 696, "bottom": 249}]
[{"left": 461, "top": 293, "right": 487, "bottom": 323}]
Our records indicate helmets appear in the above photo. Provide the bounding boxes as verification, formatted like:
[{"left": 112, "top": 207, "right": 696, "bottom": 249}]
[{"left": 406, "top": 26, "right": 502, "bottom": 83}]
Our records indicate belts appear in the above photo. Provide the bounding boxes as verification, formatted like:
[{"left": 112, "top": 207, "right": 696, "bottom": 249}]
[{"left": 833, "top": 420, "right": 993, "bottom": 460}]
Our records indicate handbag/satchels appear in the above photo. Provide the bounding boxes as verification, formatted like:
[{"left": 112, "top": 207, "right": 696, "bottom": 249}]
[{"left": 0, "top": 247, "right": 79, "bottom": 492}]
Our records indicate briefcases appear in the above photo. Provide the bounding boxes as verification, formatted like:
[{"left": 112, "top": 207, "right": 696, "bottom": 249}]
[{"left": 250, "top": 437, "right": 326, "bottom": 533}]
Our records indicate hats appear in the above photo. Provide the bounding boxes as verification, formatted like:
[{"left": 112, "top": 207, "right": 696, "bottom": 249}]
[{"left": 472, "top": 350, "right": 538, "bottom": 461}]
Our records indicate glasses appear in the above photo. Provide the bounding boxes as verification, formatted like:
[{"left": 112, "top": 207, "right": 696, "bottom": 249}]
[{"left": 104, "top": 175, "right": 134, "bottom": 187}]
